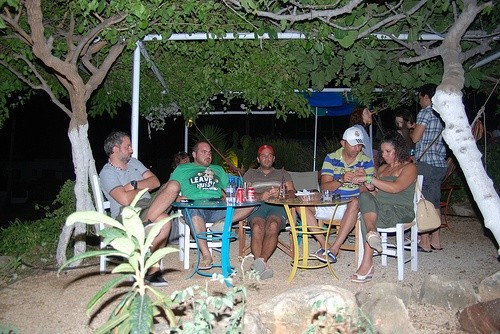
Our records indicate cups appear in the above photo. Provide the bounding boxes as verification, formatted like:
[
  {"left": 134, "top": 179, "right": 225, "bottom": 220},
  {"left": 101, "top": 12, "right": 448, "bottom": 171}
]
[{"left": 322, "top": 190, "right": 333, "bottom": 201}]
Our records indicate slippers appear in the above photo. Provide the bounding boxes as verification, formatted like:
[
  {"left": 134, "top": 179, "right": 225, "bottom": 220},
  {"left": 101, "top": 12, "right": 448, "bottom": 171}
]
[
  {"left": 193, "top": 258, "right": 213, "bottom": 270},
  {"left": 309, "top": 247, "right": 337, "bottom": 263},
  {"left": 403, "top": 242, "right": 443, "bottom": 252}
]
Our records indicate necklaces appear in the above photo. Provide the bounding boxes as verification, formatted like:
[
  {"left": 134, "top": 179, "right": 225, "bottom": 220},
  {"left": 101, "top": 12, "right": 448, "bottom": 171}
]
[{"left": 108, "top": 158, "right": 121, "bottom": 170}]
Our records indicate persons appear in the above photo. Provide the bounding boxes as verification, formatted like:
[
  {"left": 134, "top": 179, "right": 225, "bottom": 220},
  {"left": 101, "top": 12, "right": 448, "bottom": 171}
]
[
  {"left": 297, "top": 82, "right": 450, "bottom": 283},
  {"left": 101, "top": 131, "right": 298, "bottom": 281}
]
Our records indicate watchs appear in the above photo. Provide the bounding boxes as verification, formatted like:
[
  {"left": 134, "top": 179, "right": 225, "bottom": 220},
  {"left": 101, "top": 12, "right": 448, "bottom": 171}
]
[{"left": 131, "top": 180, "right": 137, "bottom": 190}]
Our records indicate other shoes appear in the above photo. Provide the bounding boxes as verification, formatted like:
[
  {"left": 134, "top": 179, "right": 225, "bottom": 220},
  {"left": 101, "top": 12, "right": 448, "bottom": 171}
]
[
  {"left": 249, "top": 257, "right": 273, "bottom": 280},
  {"left": 145, "top": 272, "right": 168, "bottom": 286},
  {"left": 209, "top": 220, "right": 225, "bottom": 233}
]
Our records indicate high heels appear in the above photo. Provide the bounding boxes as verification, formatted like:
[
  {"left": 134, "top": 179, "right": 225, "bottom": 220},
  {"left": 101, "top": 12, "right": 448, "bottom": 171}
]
[
  {"left": 366, "top": 231, "right": 383, "bottom": 253},
  {"left": 350, "top": 264, "right": 374, "bottom": 283}
]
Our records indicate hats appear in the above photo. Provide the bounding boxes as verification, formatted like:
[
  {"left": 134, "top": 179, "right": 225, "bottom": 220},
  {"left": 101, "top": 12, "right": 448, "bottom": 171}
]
[
  {"left": 258, "top": 145, "right": 273, "bottom": 154},
  {"left": 343, "top": 126, "right": 365, "bottom": 147}
]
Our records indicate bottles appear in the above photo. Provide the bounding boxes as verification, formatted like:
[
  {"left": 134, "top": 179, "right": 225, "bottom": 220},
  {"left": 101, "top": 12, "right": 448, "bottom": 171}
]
[
  {"left": 278, "top": 182, "right": 286, "bottom": 199},
  {"left": 225, "top": 177, "right": 243, "bottom": 203}
]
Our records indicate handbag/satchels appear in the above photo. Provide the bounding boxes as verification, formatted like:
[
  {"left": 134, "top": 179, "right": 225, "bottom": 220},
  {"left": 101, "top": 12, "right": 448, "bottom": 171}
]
[{"left": 415, "top": 197, "right": 441, "bottom": 234}]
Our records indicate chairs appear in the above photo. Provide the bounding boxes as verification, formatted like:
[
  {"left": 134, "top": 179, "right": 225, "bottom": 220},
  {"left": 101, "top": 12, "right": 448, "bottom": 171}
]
[
  {"left": 92, "top": 174, "right": 164, "bottom": 277},
  {"left": 356, "top": 175, "right": 425, "bottom": 281},
  {"left": 238, "top": 217, "right": 295, "bottom": 259},
  {"left": 440, "top": 184, "right": 455, "bottom": 234},
  {"left": 178, "top": 190, "right": 222, "bottom": 270},
  {"left": 316, "top": 169, "right": 359, "bottom": 270}
]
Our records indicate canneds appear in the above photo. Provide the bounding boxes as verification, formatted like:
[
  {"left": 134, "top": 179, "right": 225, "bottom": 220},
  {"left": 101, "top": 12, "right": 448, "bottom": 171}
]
[
  {"left": 247, "top": 187, "right": 255, "bottom": 201},
  {"left": 236, "top": 188, "right": 245, "bottom": 203}
]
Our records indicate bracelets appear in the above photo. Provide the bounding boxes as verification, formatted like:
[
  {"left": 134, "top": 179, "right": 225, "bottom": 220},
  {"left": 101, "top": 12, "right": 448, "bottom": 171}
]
[
  {"left": 368, "top": 187, "right": 375, "bottom": 192},
  {"left": 337, "top": 177, "right": 344, "bottom": 187},
  {"left": 366, "top": 175, "right": 375, "bottom": 184}
]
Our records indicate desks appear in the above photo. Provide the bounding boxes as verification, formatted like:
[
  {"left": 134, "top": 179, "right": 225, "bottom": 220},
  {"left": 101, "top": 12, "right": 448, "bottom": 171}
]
[
  {"left": 263, "top": 191, "right": 352, "bottom": 286},
  {"left": 170, "top": 197, "right": 261, "bottom": 289}
]
